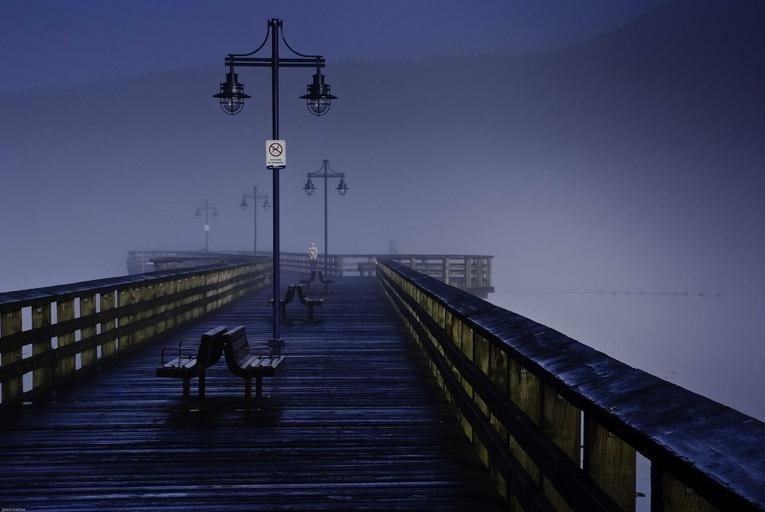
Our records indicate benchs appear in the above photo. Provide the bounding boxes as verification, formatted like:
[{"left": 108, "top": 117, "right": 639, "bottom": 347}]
[
  {"left": 155, "top": 325, "right": 287, "bottom": 415},
  {"left": 267, "top": 270, "right": 336, "bottom": 322}
]
